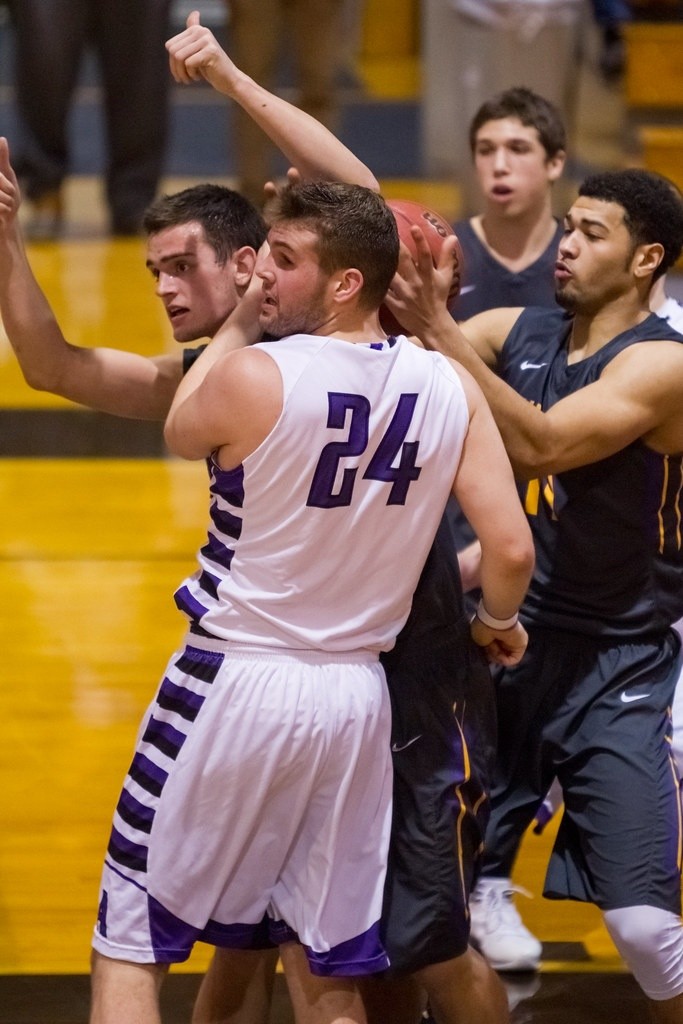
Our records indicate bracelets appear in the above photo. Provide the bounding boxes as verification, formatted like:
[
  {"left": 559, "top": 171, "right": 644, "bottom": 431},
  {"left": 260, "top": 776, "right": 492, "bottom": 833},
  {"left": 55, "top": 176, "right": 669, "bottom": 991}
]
[{"left": 476, "top": 599, "right": 519, "bottom": 630}]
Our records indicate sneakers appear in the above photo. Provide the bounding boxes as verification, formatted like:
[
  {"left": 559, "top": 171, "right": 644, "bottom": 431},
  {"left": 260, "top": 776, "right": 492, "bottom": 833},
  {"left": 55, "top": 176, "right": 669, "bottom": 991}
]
[
  {"left": 469, "top": 878, "right": 542, "bottom": 971},
  {"left": 499, "top": 969, "right": 540, "bottom": 1014}
]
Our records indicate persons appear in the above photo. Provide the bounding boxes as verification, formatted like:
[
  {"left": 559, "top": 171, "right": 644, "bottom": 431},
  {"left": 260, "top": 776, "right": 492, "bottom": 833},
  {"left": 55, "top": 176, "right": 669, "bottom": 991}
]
[
  {"left": 0, "top": 11, "right": 510, "bottom": 1024},
  {"left": 90, "top": 180, "right": 529, "bottom": 1024},
  {"left": 14, "top": 0, "right": 174, "bottom": 243},
  {"left": 232, "top": 0, "right": 339, "bottom": 211},
  {"left": 443, "top": 86, "right": 566, "bottom": 621},
  {"left": 385, "top": 169, "right": 682, "bottom": 1024}
]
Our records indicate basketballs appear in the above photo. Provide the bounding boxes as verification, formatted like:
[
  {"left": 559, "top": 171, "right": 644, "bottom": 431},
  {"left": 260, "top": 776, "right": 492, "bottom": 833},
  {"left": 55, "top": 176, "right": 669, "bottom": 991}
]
[{"left": 382, "top": 196, "right": 466, "bottom": 331}]
